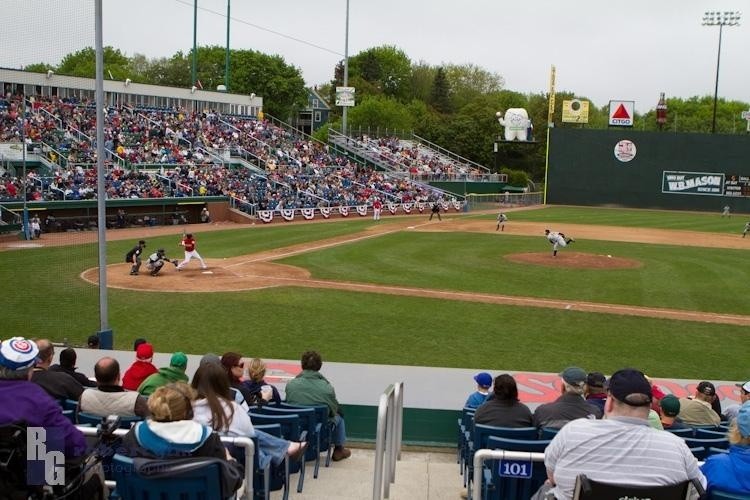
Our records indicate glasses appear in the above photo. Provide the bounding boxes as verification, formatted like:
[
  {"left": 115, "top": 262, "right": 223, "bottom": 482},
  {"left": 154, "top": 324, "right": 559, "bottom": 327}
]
[{"left": 235, "top": 363, "right": 245, "bottom": 368}]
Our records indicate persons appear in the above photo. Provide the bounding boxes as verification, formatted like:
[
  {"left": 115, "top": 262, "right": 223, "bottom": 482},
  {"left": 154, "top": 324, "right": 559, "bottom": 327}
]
[
  {"left": 126, "top": 240, "right": 146, "bottom": 275},
  {"left": 465, "top": 364, "right": 750, "bottom": 500},
  {"left": 145, "top": 249, "right": 176, "bottom": 276},
  {"left": 1, "top": 333, "right": 350, "bottom": 500},
  {"left": 1, "top": 89, "right": 529, "bottom": 239},
  {"left": 722, "top": 205, "right": 732, "bottom": 219},
  {"left": 176, "top": 232, "right": 208, "bottom": 271},
  {"left": 742, "top": 222, "right": 750, "bottom": 238},
  {"left": 544, "top": 230, "right": 575, "bottom": 258}
]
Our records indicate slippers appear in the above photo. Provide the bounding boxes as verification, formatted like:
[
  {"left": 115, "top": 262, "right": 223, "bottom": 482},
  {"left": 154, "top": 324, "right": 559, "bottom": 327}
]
[{"left": 292, "top": 441, "right": 309, "bottom": 462}]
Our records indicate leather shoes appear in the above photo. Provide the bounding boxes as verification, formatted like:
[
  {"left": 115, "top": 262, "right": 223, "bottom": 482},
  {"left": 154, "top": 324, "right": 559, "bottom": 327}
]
[{"left": 332, "top": 448, "right": 352, "bottom": 462}]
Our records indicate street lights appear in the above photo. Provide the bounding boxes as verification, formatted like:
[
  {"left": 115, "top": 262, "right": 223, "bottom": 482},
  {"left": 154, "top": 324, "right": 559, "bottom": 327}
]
[{"left": 700, "top": 9, "right": 742, "bottom": 134}]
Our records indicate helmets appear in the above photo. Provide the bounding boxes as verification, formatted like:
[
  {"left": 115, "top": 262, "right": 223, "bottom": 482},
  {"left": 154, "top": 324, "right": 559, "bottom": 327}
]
[
  {"left": 139, "top": 239, "right": 146, "bottom": 247},
  {"left": 157, "top": 248, "right": 164, "bottom": 255},
  {"left": 544, "top": 230, "right": 550, "bottom": 235},
  {"left": 185, "top": 233, "right": 193, "bottom": 239}
]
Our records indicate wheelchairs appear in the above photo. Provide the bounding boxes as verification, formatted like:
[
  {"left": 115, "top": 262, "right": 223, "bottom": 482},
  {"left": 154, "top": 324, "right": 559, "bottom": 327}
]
[{"left": 1, "top": 414, "right": 122, "bottom": 499}]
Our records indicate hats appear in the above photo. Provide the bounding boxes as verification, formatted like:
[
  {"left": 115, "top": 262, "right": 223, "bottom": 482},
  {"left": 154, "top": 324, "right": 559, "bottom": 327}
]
[
  {"left": 199, "top": 353, "right": 222, "bottom": 366},
  {"left": 695, "top": 383, "right": 717, "bottom": 395},
  {"left": 733, "top": 381, "right": 750, "bottom": 394},
  {"left": 737, "top": 401, "right": 749, "bottom": 440},
  {"left": 557, "top": 367, "right": 680, "bottom": 417},
  {"left": 135, "top": 342, "right": 154, "bottom": 360},
  {"left": 1, "top": 335, "right": 39, "bottom": 370},
  {"left": 473, "top": 372, "right": 493, "bottom": 388},
  {"left": 170, "top": 352, "right": 188, "bottom": 366},
  {"left": 87, "top": 334, "right": 101, "bottom": 346}
]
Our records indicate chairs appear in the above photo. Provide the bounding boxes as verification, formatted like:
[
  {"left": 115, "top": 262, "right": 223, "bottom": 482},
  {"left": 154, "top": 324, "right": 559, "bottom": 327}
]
[
  {"left": 1, "top": 91, "right": 457, "bottom": 216},
  {"left": 46, "top": 374, "right": 342, "bottom": 498},
  {"left": 450, "top": 401, "right": 744, "bottom": 498}
]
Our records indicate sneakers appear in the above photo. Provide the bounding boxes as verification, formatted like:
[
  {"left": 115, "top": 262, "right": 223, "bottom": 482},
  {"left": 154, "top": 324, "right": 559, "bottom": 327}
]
[
  {"left": 569, "top": 237, "right": 576, "bottom": 244},
  {"left": 460, "top": 486, "right": 473, "bottom": 499},
  {"left": 130, "top": 271, "right": 139, "bottom": 275},
  {"left": 152, "top": 272, "right": 159, "bottom": 276},
  {"left": 176, "top": 267, "right": 183, "bottom": 272}
]
